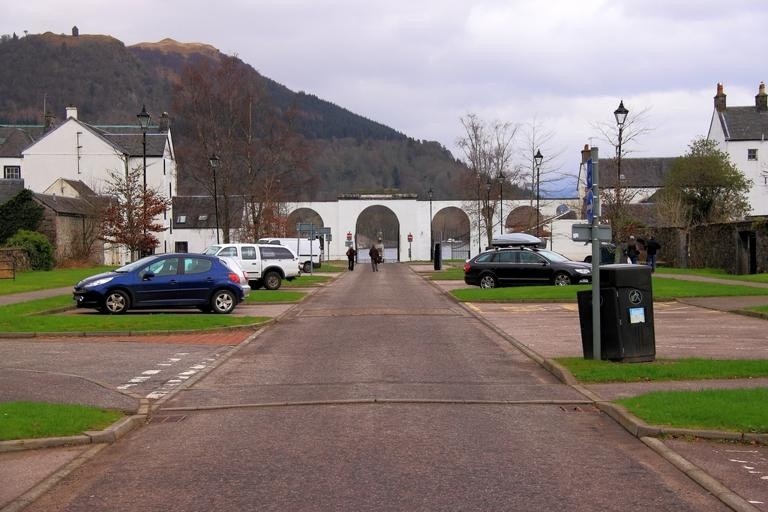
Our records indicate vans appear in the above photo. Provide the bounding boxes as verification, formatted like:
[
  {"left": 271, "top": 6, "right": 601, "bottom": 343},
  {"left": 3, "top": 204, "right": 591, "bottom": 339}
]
[{"left": 257, "top": 237, "right": 321, "bottom": 273}]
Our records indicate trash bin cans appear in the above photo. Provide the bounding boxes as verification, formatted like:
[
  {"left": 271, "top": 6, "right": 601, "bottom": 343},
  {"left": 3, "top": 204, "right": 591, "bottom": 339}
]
[
  {"left": 595, "top": 263, "right": 656, "bottom": 363},
  {"left": 599, "top": 241, "right": 616, "bottom": 264}
]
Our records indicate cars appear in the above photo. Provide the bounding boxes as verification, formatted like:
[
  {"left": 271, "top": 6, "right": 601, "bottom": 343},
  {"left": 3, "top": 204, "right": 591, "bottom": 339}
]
[
  {"left": 71, "top": 252, "right": 251, "bottom": 314},
  {"left": 464, "top": 231, "right": 592, "bottom": 290}
]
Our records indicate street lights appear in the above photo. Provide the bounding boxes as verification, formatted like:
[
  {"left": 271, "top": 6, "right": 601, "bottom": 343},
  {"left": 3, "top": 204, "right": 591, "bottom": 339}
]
[
  {"left": 486, "top": 178, "right": 492, "bottom": 247},
  {"left": 533, "top": 148, "right": 546, "bottom": 237},
  {"left": 497, "top": 171, "right": 506, "bottom": 235},
  {"left": 136, "top": 104, "right": 150, "bottom": 235},
  {"left": 428, "top": 184, "right": 434, "bottom": 262},
  {"left": 208, "top": 152, "right": 221, "bottom": 247},
  {"left": 613, "top": 99, "right": 630, "bottom": 204}
]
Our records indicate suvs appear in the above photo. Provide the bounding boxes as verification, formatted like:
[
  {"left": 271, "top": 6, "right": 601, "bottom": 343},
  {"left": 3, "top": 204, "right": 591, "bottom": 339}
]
[{"left": 199, "top": 244, "right": 300, "bottom": 290}]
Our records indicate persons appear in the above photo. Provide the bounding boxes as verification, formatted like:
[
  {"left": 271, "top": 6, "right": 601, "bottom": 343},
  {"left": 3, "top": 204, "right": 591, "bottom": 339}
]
[
  {"left": 345, "top": 245, "right": 357, "bottom": 271},
  {"left": 642, "top": 235, "right": 662, "bottom": 273},
  {"left": 367, "top": 244, "right": 380, "bottom": 272},
  {"left": 626, "top": 237, "right": 641, "bottom": 264}
]
